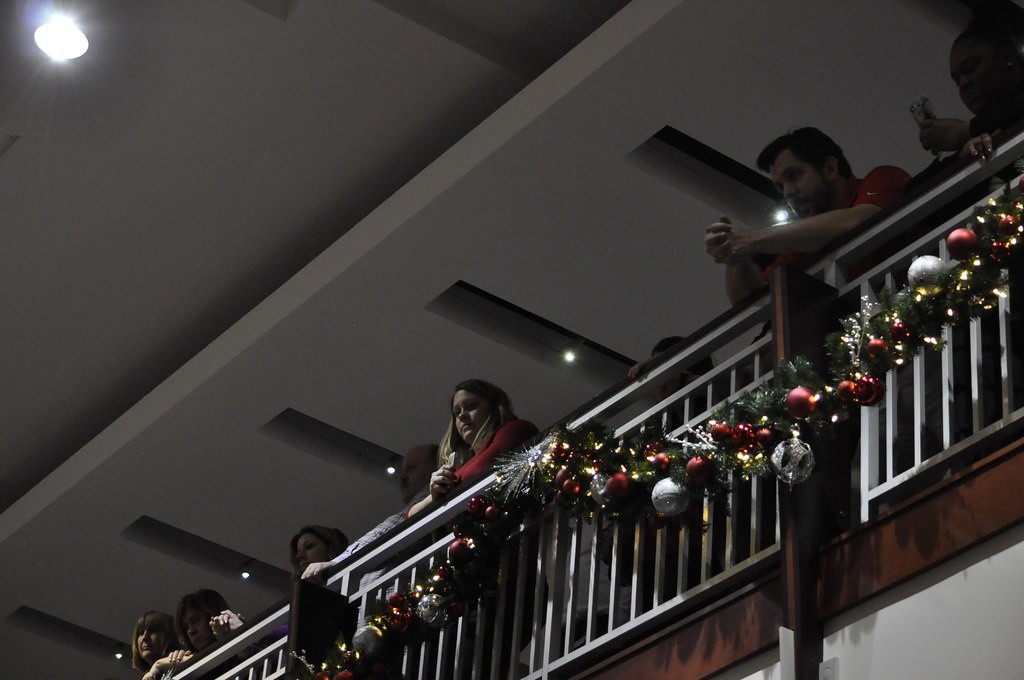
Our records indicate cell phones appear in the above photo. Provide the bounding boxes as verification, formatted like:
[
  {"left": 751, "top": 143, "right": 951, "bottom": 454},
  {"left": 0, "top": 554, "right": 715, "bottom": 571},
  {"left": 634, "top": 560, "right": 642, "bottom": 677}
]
[
  {"left": 910, "top": 94, "right": 937, "bottom": 125},
  {"left": 447, "top": 452, "right": 456, "bottom": 471}
]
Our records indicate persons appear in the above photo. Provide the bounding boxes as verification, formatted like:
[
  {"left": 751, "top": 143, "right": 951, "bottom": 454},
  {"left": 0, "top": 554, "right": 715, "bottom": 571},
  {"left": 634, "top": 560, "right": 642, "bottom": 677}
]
[
  {"left": 288, "top": 523, "right": 396, "bottom": 680},
  {"left": 625, "top": 332, "right": 748, "bottom": 586},
  {"left": 918, "top": 20, "right": 1024, "bottom": 165},
  {"left": 700, "top": 124, "right": 965, "bottom": 472},
  {"left": 396, "top": 374, "right": 541, "bottom": 680},
  {"left": 131, "top": 588, "right": 247, "bottom": 680}
]
